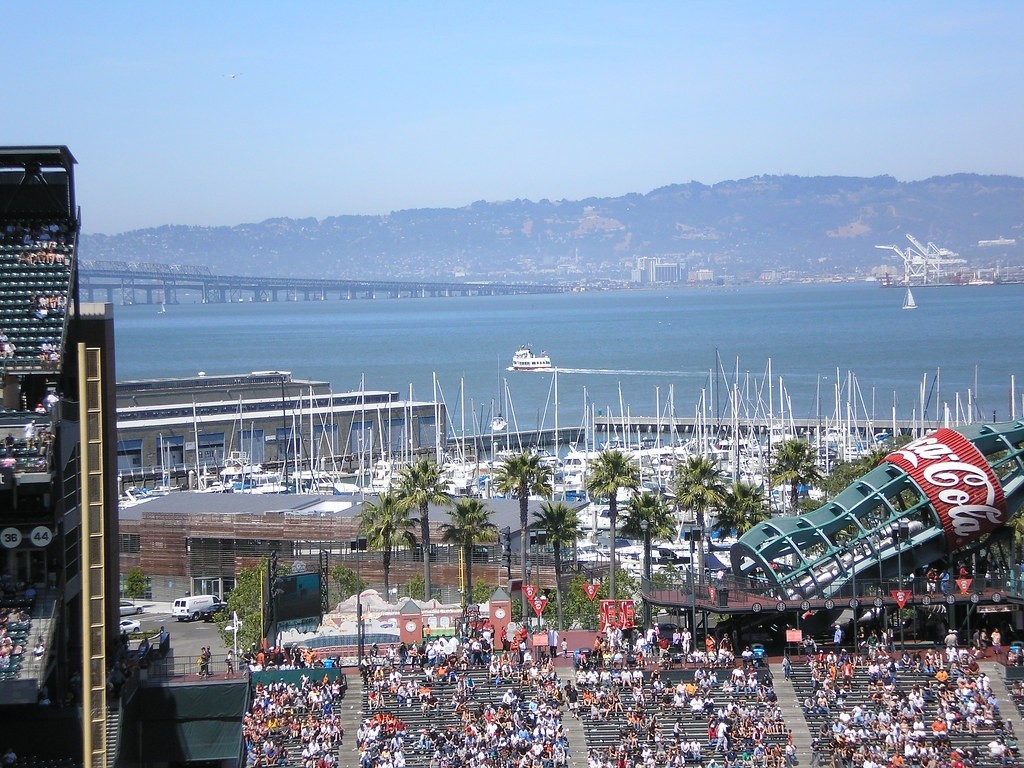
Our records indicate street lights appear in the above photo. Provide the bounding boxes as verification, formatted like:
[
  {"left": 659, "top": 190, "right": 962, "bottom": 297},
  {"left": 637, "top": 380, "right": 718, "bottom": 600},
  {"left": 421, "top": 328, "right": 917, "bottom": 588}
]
[
  {"left": 225, "top": 611, "right": 243, "bottom": 673},
  {"left": 890, "top": 522, "right": 909, "bottom": 660},
  {"left": 683, "top": 525, "right": 702, "bottom": 652}
]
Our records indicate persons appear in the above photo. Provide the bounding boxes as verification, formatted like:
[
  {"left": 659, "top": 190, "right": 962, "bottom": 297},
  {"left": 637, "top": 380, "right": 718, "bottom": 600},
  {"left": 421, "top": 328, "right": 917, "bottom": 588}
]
[{"left": 0, "top": 218, "right": 1024, "bottom": 768}]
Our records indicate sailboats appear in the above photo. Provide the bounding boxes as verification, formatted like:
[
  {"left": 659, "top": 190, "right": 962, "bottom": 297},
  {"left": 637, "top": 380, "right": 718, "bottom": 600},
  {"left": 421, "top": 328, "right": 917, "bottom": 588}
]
[
  {"left": 118, "top": 353, "right": 1015, "bottom": 579},
  {"left": 902, "top": 286, "right": 918, "bottom": 310}
]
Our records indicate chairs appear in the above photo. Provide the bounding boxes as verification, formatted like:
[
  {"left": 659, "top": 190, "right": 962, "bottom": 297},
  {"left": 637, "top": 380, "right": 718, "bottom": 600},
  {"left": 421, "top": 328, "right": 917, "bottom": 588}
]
[
  {"left": 672, "top": 655, "right": 683, "bottom": 666},
  {"left": 751, "top": 644, "right": 769, "bottom": 664}
]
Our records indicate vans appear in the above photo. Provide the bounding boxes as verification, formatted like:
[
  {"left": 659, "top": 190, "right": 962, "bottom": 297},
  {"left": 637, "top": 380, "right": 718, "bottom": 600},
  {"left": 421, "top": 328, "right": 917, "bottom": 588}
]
[{"left": 171, "top": 594, "right": 228, "bottom": 622}]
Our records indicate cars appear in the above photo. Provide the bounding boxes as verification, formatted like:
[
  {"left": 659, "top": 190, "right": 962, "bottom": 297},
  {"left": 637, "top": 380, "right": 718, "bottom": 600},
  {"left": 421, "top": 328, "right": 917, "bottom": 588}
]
[
  {"left": 119, "top": 619, "right": 141, "bottom": 635},
  {"left": 119, "top": 600, "right": 146, "bottom": 617},
  {"left": 203, "top": 601, "right": 229, "bottom": 623}
]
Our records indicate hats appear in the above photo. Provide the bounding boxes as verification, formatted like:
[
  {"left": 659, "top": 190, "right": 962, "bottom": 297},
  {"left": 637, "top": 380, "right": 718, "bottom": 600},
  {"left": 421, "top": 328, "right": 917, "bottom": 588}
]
[
  {"left": 309, "top": 647, "right": 313, "bottom": 649},
  {"left": 628, "top": 707, "right": 632, "bottom": 711},
  {"left": 829, "top": 651, "right": 833, "bottom": 654},
  {"left": 891, "top": 696, "right": 898, "bottom": 701},
  {"left": 841, "top": 649, "right": 846, "bottom": 651},
  {"left": 531, "top": 697, "right": 536, "bottom": 700},
  {"left": 638, "top": 633, "right": 642, "bottom": 637},
  {"left": 541, "top": 628, "right": 547, "bottom": 631},
  {"left": 388, "top": 720, "right": 393, "bottom": 725},
  {"left": 280, "top": 678, "right": 284, "bottom": 680},
  {"left": 834, "top": 625, "right": 840, "bottom": 628},
  {"left": 819, "top": 650, "right": 823, "bottom": 653},
  {"left": 481, "top": 747, "right": 485, "bottom": 749},
  {"left": 429, "top": 728, "right": 435, "bottom": 732},
  {"left": 939, "top": 667, "right": 944, "bottom": 669}
]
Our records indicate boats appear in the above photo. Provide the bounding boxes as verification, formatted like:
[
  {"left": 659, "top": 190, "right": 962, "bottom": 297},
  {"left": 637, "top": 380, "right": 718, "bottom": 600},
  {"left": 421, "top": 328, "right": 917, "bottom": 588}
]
[{"left": 512, "top": 341, "right": 552, "bottom": 369}]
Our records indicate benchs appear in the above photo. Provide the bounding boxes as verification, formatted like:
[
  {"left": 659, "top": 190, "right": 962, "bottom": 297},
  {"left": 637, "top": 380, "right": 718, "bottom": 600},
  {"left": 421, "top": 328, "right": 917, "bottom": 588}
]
[
  {"left": 788, "top": 660, "right": 1024, "bottom": 768},
  {"left": 245, "top": 686, "right": 343, "bottom": 768},
  {"left": 575, "top": 680, "right": 789, "bottom": 768},
  {"left": 361, "top": 665, "right": 562, "bottom": 768}
]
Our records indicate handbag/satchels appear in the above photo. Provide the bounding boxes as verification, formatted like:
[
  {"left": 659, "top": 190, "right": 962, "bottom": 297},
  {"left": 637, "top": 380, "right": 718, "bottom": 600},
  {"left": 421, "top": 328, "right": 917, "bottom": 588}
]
[
  {"left": 789, "top": 664, "right": 792, "bottom": 671},
  {"left": 225, "top": 659, "right": 230, "bottom": 663},
  {"left": 689, "top": 639, "right": 692, "bottom": 645},
  {"left": 644, "top": 645, "right": 648, "bottom": 651},
  {"left": 197, "top": 656, "right": 202, "bottom": 666}
]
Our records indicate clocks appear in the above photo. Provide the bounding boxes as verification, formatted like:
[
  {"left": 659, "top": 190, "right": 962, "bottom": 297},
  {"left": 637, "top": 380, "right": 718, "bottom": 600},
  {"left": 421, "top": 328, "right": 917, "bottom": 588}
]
[
  {"left": 495, "top": 609, "right": 506, "bottom": 619},
  {"left": 405, "top": 621, "right": 417, "bottom": 632}
]
